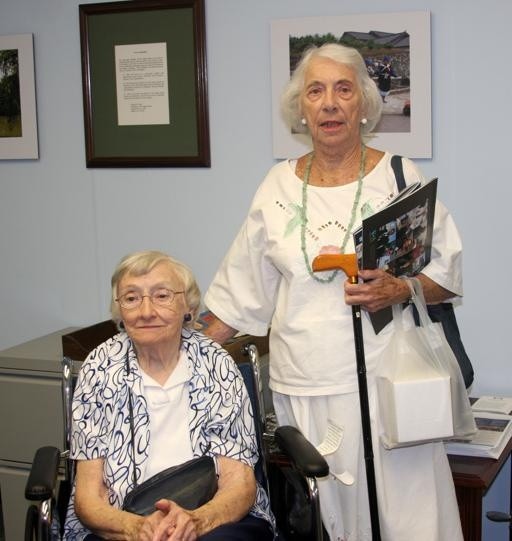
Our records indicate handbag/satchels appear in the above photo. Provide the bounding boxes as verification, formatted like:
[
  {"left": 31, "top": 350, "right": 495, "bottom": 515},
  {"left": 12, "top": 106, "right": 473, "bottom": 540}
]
[
  {"left": 368, "top": 154, "right": 475, "bottom": 388},
  {"left": 122, "top": 455, "right": 219, "bottom": 516}
]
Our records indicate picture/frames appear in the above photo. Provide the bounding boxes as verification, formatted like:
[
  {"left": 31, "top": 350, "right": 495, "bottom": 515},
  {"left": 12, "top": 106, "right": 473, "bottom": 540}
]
[
  {"left": 269, "top": 10, "right": 434, "bottom": 161},
  {"left": 0, "top": 31, "right": 40, "bottom": 159},
  {"left": 77, "top": 0, "right": 212, "bottom": 168}
]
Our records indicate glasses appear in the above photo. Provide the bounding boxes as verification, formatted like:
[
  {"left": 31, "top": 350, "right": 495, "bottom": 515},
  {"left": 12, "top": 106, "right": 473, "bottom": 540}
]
[{"left": 114, "top": 286, "right": 185, "bottom": 309}]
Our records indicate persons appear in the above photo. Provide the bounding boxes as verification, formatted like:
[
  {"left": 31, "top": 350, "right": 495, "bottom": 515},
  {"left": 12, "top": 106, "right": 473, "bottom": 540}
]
[
  {"left": 201, "top": 44, "right": 466, "bottom": 540},
  {"left": 64, "top": 253, "right": 277, "bottom": 540},
  {"left": 373, "top": 202, "right": 426, "bottom": 275},
  {"left": 363, "top": 54, "right": 395, "bottom": 104}
]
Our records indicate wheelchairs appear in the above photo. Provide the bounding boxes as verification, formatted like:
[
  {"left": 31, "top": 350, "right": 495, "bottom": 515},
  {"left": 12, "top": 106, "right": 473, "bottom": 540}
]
[{"left": 25, "top": 343, "right": 330, "bottom": 541}]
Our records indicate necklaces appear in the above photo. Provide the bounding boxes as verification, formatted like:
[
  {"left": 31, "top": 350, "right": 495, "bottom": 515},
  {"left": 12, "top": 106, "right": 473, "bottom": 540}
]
[{"left": 299, "top": 140, "right": 367, "bottom": 284}]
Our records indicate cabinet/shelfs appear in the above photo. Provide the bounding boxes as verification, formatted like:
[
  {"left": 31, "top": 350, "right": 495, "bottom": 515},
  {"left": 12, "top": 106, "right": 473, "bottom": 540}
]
[{"left": 1, "top": 327, "right": 274, "bottom": 540}]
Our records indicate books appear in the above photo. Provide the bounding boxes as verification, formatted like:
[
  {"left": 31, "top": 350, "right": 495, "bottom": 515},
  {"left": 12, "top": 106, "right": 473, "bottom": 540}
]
[
  {"left": 441, "top": 395, "right": 511, "bottom": 461},
  {"left": 352, "top": 174, "right": 438, "bottom": 334}
]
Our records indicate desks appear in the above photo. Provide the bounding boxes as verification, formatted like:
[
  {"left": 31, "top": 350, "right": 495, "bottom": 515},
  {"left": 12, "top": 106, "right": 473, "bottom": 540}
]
[{"left": 446, "top": 396, "right": 511, "bottom": 539}]
